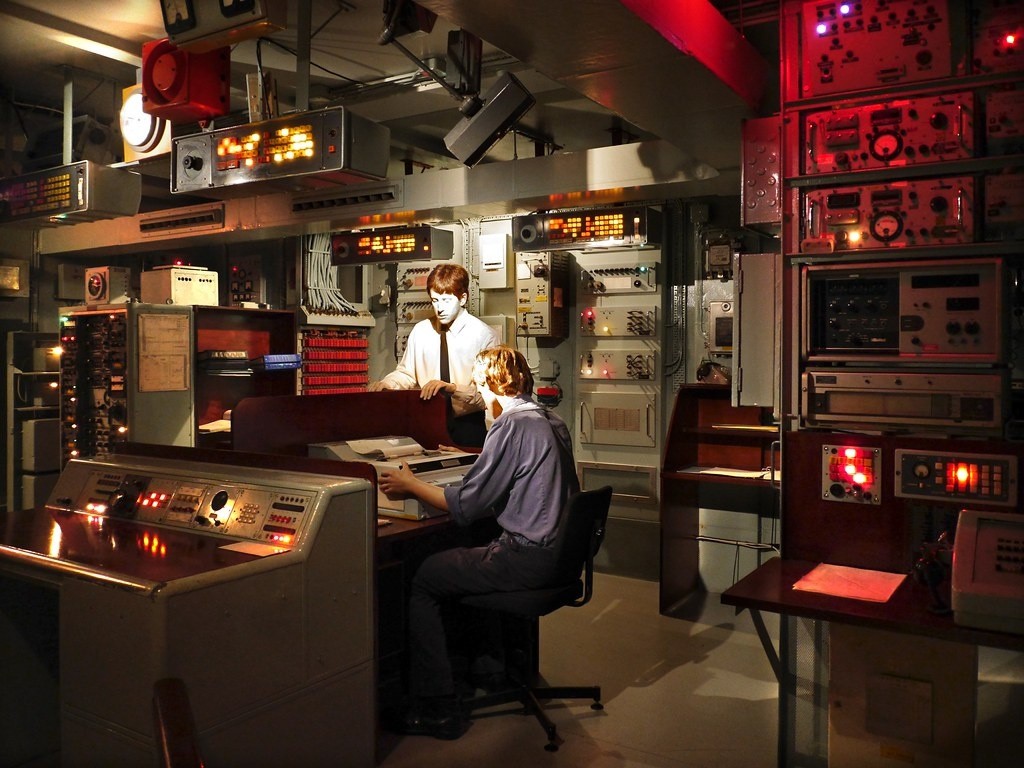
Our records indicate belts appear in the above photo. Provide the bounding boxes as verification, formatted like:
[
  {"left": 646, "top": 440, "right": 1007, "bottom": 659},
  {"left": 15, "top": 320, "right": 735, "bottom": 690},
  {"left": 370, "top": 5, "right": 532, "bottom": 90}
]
[{"left": 500, "top": 528, "right": 549, "bottom": 553}]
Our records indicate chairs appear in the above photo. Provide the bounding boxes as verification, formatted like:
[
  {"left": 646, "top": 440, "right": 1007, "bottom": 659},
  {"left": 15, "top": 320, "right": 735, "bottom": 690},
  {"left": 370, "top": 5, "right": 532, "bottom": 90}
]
[{"left": 399, "top": 485, "right": 614, "bottom": 752}]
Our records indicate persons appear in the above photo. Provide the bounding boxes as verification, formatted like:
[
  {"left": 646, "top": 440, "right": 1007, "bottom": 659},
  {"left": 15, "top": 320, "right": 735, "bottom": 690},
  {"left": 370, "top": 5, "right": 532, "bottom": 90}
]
[
  {"left": 378, "top": 350, "right": 582, "bottom": 740},
  {"left": 367, "top": 264, "right": 502, "bottom": 456}
]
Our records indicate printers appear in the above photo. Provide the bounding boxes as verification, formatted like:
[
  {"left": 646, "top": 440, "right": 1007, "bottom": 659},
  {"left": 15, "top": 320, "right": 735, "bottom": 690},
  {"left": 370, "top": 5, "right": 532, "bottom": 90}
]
[{"left": 307, "top": 435, "right": 479, "bottom": 521}]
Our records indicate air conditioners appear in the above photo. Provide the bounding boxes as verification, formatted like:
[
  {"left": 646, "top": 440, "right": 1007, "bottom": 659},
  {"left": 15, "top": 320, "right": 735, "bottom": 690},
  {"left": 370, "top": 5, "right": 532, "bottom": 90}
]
[
  {"left": 139, "top": 201, "right": 226, "bottom": 239},
  {"left": 286, "top": 176, "right": 404, "bottom": 220}
]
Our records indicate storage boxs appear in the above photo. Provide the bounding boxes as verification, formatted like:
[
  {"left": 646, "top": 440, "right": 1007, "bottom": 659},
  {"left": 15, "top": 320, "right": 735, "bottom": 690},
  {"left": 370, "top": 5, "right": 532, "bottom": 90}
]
[{"left": 197, "top": 330, "right": 270, "bottom": 362}]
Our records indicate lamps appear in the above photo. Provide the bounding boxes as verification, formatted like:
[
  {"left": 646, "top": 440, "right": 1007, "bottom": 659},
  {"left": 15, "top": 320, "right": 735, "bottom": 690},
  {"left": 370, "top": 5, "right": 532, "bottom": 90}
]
[{"left": 370, "top": 0, "right": 534, "bottom": 164}]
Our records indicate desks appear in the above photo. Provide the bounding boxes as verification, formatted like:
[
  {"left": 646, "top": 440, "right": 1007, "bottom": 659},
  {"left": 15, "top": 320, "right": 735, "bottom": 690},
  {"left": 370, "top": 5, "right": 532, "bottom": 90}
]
[{"left": 720, "top": 556, "right": 1024, "bottom": 768}]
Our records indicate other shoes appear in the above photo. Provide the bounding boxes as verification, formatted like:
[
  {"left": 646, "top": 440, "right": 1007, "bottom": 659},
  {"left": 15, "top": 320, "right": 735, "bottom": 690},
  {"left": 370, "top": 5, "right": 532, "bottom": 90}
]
[{"left": 386, "top": 707, "right": 470, "bottom": 741}]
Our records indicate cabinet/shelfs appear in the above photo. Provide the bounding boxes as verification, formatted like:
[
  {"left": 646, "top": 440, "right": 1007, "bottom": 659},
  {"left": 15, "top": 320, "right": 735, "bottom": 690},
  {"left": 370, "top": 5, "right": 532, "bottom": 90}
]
[{"left": 7, "top": 330, "right": 60, "bottom": 512}]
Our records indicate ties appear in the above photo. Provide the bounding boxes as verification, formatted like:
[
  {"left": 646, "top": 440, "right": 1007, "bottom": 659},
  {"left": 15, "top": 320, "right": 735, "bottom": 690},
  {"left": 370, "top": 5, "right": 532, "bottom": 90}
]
[{"left": 440, "top": 329, "right": 451, "bottom": 383}]
